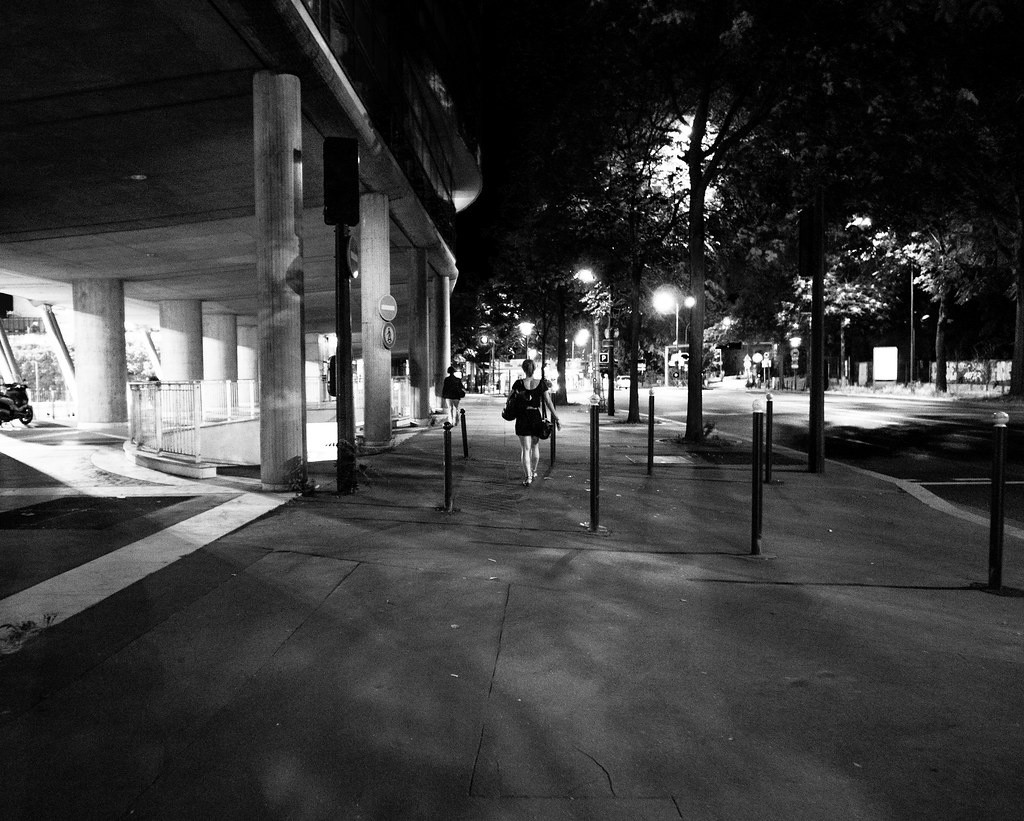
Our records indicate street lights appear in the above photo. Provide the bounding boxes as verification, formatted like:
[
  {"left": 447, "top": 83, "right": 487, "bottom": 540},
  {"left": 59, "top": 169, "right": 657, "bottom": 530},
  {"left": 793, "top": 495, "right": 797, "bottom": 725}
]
[
  {"left": 656, "top": 292, "right": 694, "bottom": 346},
  {"left": 519, "top": 321, "right": 534, "bottom": 359}
]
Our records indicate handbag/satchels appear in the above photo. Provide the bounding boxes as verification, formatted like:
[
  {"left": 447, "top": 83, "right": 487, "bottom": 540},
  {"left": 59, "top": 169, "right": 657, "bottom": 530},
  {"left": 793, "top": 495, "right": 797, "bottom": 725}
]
[
  {"left": 540, "top": 419, "right": 552, "bottom": 438},
  {"left": 457, "top": 380, "right": 465, "bottom": 399},
  {"left": 502, "top": 381, "right": 528, "bottom": 421}
]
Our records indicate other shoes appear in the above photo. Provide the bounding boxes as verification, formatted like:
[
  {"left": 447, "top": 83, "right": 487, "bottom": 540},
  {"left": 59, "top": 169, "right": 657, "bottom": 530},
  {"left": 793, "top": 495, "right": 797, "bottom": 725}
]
[{"left": 523, "top": 469, "right": 537, "bottom": 486}]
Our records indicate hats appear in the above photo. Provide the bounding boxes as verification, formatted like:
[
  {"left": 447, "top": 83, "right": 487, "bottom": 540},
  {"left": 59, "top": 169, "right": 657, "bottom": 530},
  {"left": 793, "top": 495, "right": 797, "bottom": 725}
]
[{"left": 448, "top": 367, "right": 457, "bottom": 372}]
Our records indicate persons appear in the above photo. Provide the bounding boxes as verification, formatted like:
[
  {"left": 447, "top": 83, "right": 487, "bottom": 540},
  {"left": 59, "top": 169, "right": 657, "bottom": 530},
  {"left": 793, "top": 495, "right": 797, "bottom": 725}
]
[
  {"left": 442, "top": 366, "right": 466, "bottom": 426},
  {"left": 504, "top": 359, "right": 562, "bottom": 486}
]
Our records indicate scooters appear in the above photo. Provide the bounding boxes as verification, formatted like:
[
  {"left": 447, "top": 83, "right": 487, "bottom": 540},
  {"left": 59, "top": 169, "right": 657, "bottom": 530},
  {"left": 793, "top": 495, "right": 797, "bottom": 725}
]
[{"left": 0, "top": 378, "right": 34, "bottom": 426}]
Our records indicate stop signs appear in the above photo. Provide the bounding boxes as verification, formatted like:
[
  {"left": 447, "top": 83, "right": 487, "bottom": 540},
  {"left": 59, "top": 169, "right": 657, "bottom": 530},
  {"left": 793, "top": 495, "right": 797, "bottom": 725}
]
[
  {"left": 343, "top": 237, "right": 359, "bottom": 278},
  {"left": 379, "top": 294, "right": 397, "bottom": 322}
]
